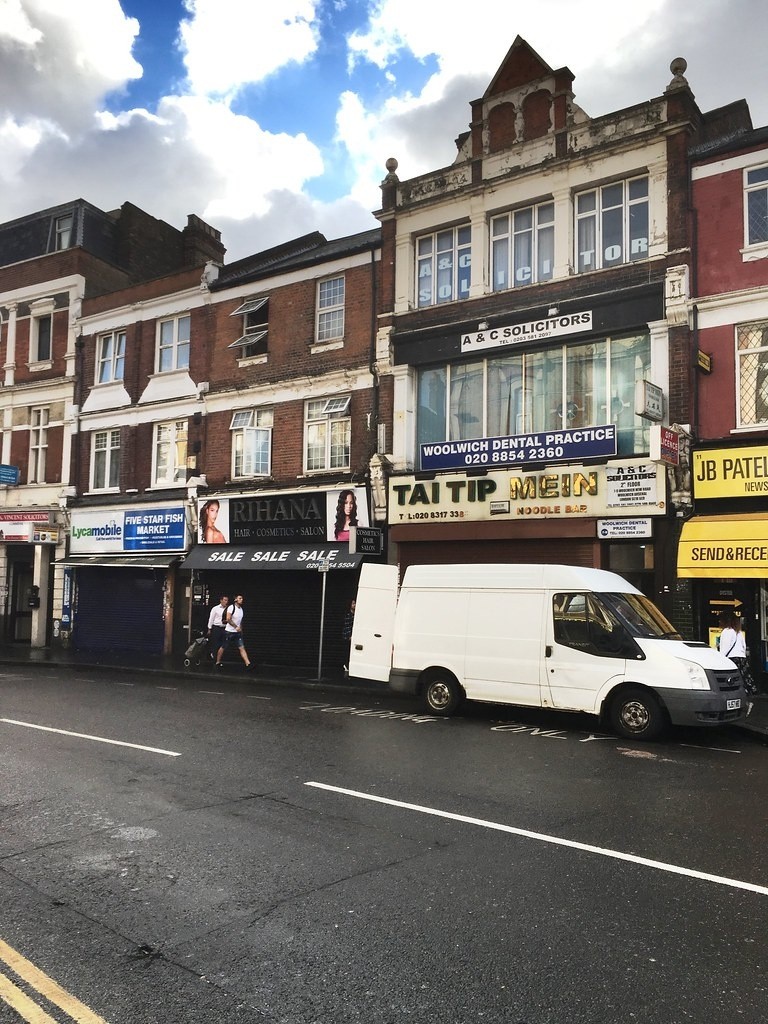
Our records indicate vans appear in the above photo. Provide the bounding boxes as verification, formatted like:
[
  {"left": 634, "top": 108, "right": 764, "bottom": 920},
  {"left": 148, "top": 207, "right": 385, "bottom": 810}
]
[{"left": 349, "top": 563, "right": 749, "bottom": 744}]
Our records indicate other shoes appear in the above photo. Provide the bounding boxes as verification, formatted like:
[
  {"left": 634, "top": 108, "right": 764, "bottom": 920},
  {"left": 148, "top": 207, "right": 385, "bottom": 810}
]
[
  {"left": 746, "top": 702, "right": 755, "bottom": 717},
  {"left": 244, "top": 663, "right": 257, "bottom": 671},
  {"left": 215, "top": 662, "right": 224, "bottom": 668},
  {"left": 343, "top": 665, "right": 349, "bottom": 672}
]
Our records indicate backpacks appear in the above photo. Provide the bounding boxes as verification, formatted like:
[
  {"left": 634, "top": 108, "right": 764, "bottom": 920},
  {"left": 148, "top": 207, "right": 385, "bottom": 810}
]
[{"left": 222, "top": 604, "right": 235, "bottom": 624}]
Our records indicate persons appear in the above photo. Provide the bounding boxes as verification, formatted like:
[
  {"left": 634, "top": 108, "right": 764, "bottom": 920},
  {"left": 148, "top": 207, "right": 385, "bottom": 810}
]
[
  {"left": 199, "top": 500, "right": 229, "bottom": 544},
  {"left": 205, "top": 594, "right": 255, "bottom": 672},
  {"left": 332, "top": 490, "right": 359, "bottom": 541},
  {"left": 718, "top": 610, "right": 755, "bottom": 719}
]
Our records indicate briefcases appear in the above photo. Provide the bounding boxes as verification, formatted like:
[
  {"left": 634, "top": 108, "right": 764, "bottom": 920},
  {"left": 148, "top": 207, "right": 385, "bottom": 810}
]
[{"left": 183, "top": 635, "right": 209, "bottom": 667}]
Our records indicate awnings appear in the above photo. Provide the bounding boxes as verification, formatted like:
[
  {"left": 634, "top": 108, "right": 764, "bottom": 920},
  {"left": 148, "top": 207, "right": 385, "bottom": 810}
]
[
  {"left": 676, "top": 511, "right": 768, "bottom": 579},
  {"left": 178, "top": 544, "right": 364, "bottom": 570},
  {"left": 48, "top": 552, "right": 187, "bottom": 569}
]
